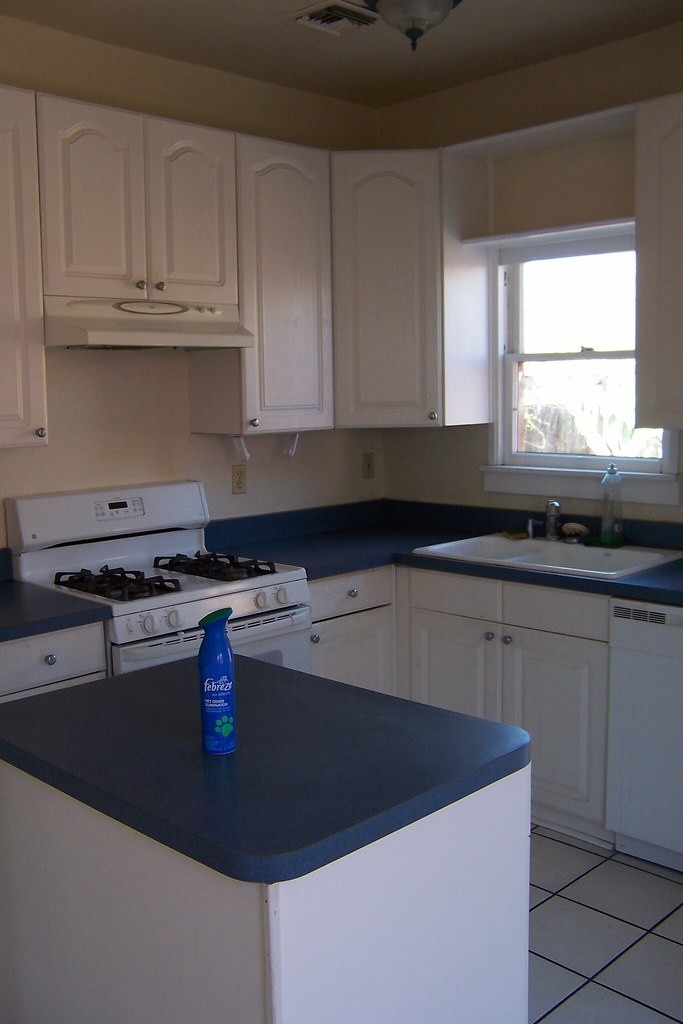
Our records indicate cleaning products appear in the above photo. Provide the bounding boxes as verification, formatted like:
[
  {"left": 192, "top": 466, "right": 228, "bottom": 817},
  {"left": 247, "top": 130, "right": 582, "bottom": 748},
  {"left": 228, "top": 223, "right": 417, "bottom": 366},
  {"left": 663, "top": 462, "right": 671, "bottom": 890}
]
[{"left": 600, "top": 463, "right": 626, "bottom": 546}]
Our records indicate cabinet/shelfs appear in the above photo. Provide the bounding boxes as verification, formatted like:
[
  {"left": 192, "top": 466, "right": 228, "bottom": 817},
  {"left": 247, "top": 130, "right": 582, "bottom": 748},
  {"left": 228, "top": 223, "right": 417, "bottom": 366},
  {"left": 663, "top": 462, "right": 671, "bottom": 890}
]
[
  {"left": 192, "top": 132, "right": 334, "bottom": 438},
  {"left": 0, "top": 83, "right": 51, "bottom": 449},
  {"left": 309, "top": 562, "right": 398, "bottom": 704},
  {"left": 34, "top": 89, "right": 239, "bottom": 306},
  {"left": 0, "top": 619, "right": 107, "bottom": 705},
  {"left": 394, "top": 568, "right": 613, "bottom": 840},
  {"left": 331, "top": 149, "right": 445, "bottom": 429},
  {"left": 440, "top": 91, "right": 683, "bottom": 429}
]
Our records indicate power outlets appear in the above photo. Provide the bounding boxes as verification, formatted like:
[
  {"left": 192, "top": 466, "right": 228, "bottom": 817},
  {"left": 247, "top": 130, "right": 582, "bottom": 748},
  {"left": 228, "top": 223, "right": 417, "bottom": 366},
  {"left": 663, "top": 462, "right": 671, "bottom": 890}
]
[{"left": 231, "top": 464, "right": 247, "bottom": 494}]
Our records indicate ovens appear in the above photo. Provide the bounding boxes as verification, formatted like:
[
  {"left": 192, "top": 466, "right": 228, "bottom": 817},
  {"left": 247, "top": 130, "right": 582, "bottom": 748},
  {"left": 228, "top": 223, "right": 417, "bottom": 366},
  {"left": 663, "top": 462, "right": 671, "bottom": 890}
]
[{"left": 111, "top": 604, "right": 316, "bottom": 677}]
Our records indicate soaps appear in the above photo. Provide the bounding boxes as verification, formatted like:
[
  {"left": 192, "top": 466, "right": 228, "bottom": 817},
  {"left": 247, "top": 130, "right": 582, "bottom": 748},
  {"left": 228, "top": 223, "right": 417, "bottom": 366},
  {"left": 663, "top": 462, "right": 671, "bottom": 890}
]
[{"left": 562, "top": 522, "right": 589, "bottom": 536}]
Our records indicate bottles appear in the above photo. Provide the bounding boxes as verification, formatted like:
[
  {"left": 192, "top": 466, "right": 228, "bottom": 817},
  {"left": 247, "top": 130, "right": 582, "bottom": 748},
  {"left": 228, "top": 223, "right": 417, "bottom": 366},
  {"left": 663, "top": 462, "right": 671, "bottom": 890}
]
[{"left": 601, "top": 463, "right": 625, "bottom": 547}]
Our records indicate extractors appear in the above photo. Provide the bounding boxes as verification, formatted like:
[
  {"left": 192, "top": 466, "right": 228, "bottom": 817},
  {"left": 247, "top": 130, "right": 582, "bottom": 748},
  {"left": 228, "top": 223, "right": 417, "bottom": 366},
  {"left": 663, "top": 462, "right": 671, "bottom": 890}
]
[{"left": 43, "top": 294, "right": 257, "bottom": 349}]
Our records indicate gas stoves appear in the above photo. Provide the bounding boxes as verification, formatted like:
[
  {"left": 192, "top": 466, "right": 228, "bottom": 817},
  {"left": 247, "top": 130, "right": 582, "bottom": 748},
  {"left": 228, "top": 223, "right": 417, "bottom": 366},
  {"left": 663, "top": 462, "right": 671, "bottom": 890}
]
[{"left": 2, "top": 479, "right": 310, "bottom": 646}]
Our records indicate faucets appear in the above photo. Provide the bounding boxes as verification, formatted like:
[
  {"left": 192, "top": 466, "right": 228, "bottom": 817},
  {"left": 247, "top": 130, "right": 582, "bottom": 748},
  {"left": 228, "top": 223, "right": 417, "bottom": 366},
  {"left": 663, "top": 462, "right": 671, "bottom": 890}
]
[{"left": 545, "top": 499, "right": 562, "bottom": 541}]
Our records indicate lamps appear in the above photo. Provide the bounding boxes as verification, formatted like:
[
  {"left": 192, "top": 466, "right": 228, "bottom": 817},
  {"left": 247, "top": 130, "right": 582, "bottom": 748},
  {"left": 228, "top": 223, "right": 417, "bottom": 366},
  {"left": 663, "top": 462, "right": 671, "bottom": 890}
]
[{"left": 363, "top": 1, "right": 462, "bottom": 51}]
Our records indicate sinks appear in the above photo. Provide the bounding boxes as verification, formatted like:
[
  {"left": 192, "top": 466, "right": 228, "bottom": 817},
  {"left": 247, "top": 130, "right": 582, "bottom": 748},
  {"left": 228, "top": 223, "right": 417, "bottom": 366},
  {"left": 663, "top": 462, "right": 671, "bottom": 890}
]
[{"left": 411, "top": 529, "right": 683, "bottom": 579}]
